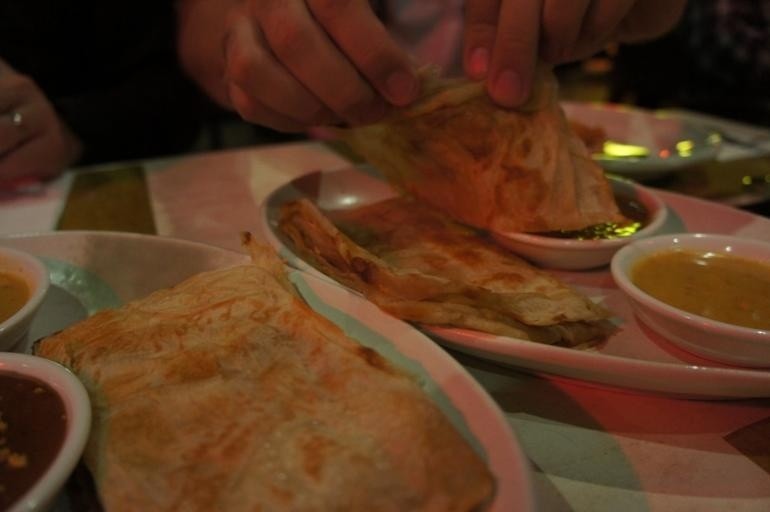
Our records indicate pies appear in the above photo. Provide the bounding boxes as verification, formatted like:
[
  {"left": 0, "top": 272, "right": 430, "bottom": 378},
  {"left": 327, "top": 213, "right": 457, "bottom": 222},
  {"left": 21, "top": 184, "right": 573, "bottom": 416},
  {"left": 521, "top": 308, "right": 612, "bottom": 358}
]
[
  {"left": 305, "top": 71, "right": 622, "bottom": 236},
  {"left": 280, "top": 191, "right": 615, "bottom": 348},
  {"left": 33, "top": 236, "right": 497, "bottom": 512}
]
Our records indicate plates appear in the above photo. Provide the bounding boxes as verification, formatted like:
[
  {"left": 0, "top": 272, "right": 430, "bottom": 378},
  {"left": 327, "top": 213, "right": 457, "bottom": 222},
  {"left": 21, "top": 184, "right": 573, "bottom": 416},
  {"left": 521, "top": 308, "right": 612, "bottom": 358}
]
[
  {"left": 2, "top": 240, "right": 54, "bottom": 345},
  {"left": 261, "top": 164, "right": 769, "bottom": 406},
  {"left": 1, "top": 230, "right": 536, "bottom": 512},
  {"left": 553, "top": 98, "right": 770, "bottom": 200},
  {"left": 1, "top": 350, "right": 92, "bottom": 512}
]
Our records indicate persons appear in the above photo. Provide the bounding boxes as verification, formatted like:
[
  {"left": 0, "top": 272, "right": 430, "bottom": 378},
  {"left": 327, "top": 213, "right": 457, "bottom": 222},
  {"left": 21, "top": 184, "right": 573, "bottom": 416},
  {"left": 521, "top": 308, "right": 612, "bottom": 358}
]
[
  {"left": 0, "top": 58, "right": 82, "bottom": 188},
  {"left": 177, "top": 1, "right": 691, "bottom": 134}
]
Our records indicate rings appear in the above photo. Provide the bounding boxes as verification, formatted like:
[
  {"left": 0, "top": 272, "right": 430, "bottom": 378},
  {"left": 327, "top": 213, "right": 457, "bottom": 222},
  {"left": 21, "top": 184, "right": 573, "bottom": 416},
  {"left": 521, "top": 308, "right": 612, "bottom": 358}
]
[{"left": 6, "top": 111, "right": 29, "bottom": 142}]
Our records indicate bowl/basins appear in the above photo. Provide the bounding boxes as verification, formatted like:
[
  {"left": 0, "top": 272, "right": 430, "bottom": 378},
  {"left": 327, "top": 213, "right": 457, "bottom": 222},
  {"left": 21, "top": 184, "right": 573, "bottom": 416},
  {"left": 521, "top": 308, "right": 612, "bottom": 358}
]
[
  {"left": 486, "top": 170, "right": 668, "bottom": 273},
  {"left": 609, "top": 232, "right": 768, "bottom": 371}
]
